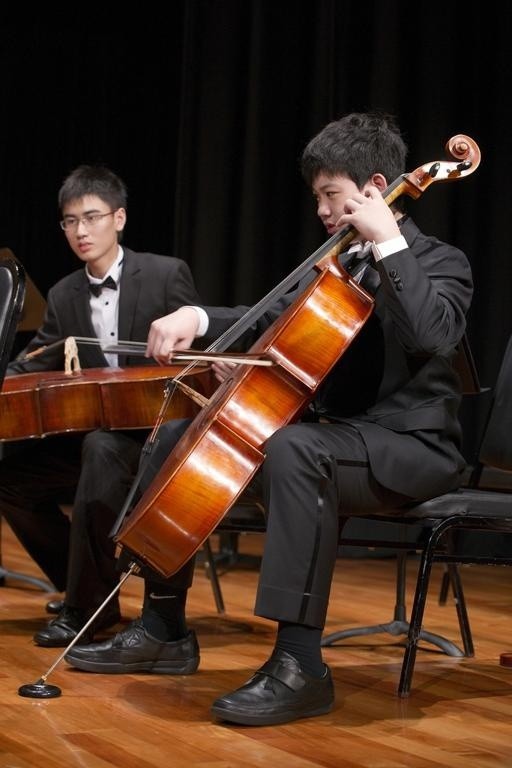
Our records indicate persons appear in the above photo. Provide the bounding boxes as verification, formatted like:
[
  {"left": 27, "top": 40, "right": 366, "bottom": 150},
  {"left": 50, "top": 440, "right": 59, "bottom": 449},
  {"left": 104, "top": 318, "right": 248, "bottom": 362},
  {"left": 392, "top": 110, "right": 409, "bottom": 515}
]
[
  {"left": 1, "top": 166, "right": 199, "bottom": 647},
  {"left": 64, "top": 113, "right": 477, "bottom": 727}
]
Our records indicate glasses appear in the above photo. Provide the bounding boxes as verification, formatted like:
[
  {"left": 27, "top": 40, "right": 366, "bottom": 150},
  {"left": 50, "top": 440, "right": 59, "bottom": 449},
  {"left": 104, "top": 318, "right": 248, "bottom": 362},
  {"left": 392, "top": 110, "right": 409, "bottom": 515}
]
[{"left": 60, "top": 213, "right": 115, "bottom": 231}]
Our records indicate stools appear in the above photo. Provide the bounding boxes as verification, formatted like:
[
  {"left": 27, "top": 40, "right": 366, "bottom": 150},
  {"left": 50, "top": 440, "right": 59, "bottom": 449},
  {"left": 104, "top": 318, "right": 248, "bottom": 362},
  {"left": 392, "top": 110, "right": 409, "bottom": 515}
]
[{"left": 202, "top": 496, "right": 269, "bottom": 618}]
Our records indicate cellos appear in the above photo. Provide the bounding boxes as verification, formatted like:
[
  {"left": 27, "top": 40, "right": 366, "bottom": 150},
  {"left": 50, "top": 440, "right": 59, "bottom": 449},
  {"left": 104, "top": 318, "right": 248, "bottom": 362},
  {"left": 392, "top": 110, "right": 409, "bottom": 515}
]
[
  {"left": 0, "top": 363, "right": 223, "bottom": 446},
  {"left": 110, "top": 131, "right": 482, "bottom": 579}
]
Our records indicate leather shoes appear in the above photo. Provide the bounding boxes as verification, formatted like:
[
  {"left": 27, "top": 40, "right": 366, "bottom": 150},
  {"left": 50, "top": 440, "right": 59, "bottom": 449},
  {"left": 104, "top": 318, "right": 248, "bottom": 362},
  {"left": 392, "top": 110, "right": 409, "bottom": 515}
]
[
  {"left": 65, "top": 613, "right": 201, "bottom": 672},
  {"left": 211, "top": 650, "right": 335, "bottom": 724},
  {"left": 33, "top": 599, "right": 121, "bottom": 648}
]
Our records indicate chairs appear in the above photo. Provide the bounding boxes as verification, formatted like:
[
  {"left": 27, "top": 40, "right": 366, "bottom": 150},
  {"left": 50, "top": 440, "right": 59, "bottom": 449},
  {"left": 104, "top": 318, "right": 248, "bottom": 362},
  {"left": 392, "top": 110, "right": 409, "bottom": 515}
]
[{"left": 339, "top": 336, "right": 512, "bottom": 697}]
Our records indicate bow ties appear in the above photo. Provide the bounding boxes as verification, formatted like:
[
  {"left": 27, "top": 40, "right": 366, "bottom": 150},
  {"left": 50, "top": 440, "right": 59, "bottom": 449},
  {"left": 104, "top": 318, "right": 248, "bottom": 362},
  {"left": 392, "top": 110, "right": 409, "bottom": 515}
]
[{"left": 89, "top": 276, "right": 117, "bottom": 297}]
